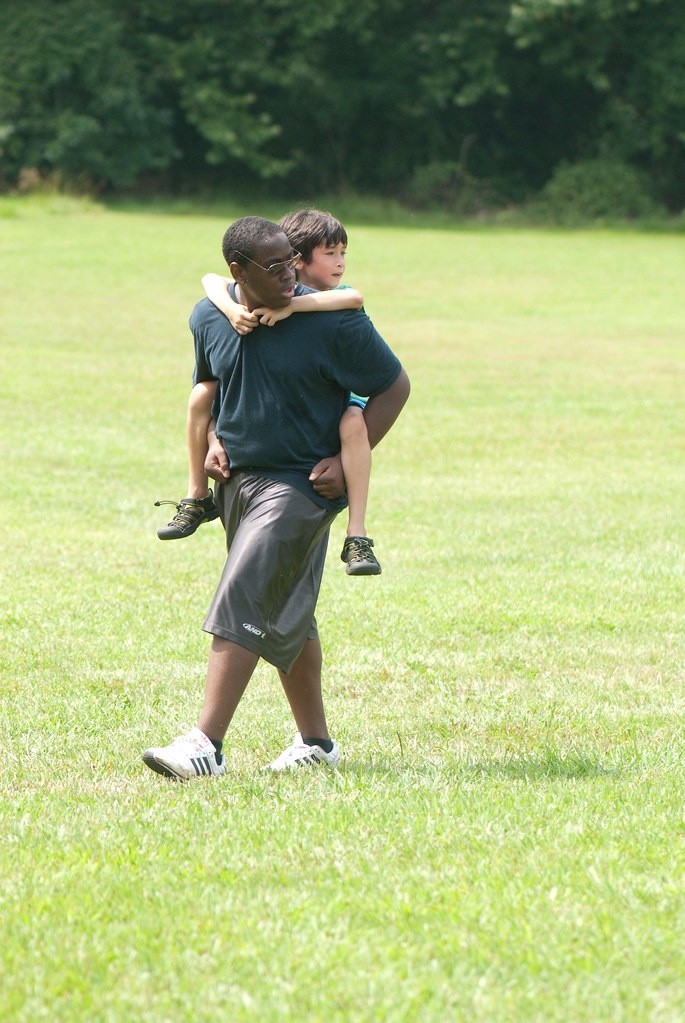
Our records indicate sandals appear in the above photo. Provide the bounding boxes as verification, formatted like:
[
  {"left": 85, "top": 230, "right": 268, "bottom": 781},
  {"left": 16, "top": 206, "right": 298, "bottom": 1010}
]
[
  {"left": 155, "top": 488, "right": 220, "bottom": 539},
  {"left": 341, "top": 536, "right": 382, "bottom": 576}
]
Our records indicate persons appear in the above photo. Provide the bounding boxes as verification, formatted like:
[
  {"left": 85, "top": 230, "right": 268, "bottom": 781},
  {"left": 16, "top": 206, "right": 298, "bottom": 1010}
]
[
  {"left": 143, "top": 216, "right": 411, "bottom": 779},
  {"left": 155, "top": 210, "right": 382, "bottom": 576}
]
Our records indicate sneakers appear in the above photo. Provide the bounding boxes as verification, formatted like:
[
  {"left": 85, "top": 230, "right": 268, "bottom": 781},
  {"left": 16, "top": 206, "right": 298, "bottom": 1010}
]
[
  {"left": 142, "top": 727, "right": 226, "bottom": 781},
  {"left": 258, "top": 732, "right": 340, "bottom": 772}
]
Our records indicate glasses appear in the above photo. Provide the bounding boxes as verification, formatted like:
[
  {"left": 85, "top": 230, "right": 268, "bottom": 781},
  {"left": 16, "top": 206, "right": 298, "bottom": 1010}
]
[{"left": 231, "top": 245, "right": 302, "bottom": 274}]
